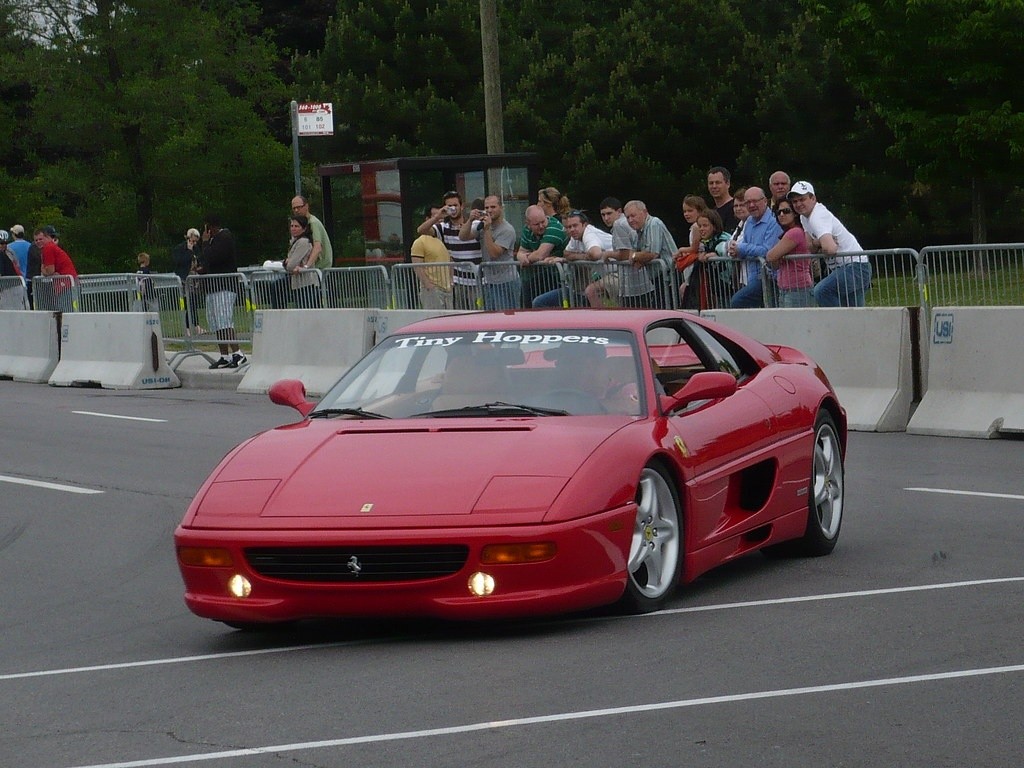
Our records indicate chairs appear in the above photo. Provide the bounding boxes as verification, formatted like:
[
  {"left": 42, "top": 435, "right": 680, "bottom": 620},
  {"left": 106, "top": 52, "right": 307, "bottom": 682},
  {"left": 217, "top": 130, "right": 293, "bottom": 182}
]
[
  {"left": 610, "top": 355, "right": 671, "bottom": 399},
  {"left": 430, "top": 352, "right": 514, "bottom": 408}
]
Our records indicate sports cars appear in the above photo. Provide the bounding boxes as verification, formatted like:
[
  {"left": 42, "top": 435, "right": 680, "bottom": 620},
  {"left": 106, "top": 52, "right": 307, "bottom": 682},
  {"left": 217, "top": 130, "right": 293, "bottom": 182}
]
[{"left": 173, "top": 308, "right": 848, "bottom": 628}]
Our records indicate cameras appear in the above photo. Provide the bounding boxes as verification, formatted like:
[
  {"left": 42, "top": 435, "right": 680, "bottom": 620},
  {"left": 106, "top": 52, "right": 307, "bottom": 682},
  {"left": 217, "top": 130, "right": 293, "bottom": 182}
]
[
  {"left": 447, "top": 207, "right": 456, "bottom": 214},
  {"left": 479, "top": 210, "right": 488, "bottom": 217}
]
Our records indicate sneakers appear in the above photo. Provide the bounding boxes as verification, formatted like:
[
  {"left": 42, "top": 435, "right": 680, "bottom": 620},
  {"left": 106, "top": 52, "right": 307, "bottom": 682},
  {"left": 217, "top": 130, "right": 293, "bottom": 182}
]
[
  {"left": 209, "top": 357, "right": 232, "bottom": 370},
  {"left": 229, "top": 353, "right": 247, "bottom": 369}
]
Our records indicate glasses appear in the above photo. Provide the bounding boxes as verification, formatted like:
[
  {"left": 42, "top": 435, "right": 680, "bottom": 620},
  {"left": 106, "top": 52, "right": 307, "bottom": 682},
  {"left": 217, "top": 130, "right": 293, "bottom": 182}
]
[
  {"left": 567, "top": 211, "right": 587, "bottom": 221},
  {"left": 775, "top": 208, "right": 797, "bottom": 216},
  {"left": 292, "top": 203, "right": 307, "bottom": 211},
  {"left": 743, "top": 197, "right": 765, "bottom": 208}
]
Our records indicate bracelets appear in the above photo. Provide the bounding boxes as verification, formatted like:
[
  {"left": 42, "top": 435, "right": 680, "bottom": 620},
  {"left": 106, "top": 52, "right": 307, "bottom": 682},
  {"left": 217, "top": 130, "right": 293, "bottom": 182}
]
[
  {"left": 631, "top": 253, "right": 637, "bottom": 262},
  {"left": 450, "top": 282, "right": 455, "bottom": 286},
  {"left": 303, "top": 264, "right": 308, "bottom": 268}
]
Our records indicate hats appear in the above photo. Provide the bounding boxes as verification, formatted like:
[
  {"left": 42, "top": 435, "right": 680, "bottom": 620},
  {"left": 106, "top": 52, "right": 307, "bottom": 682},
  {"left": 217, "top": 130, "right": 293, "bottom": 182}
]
[
  {"left": 10, "top": 225, "right": 24, "bottom": 235},
  {"left": 0, "top": 230, "right": 8, "bottom": 240},
  {"left": 786, "top": 181, "right": 814, "bottom": 200}
]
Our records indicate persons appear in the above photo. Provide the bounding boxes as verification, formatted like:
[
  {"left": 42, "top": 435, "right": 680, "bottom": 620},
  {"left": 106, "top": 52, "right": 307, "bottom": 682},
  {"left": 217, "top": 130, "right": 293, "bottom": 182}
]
[
  {"left": 459, "top": 194, "right": 520, "bottom": 310},
  {"left": 170, "top": 226, "right": 207, "bottom": 337},
  {"left": 671, "top": 193, "right": 706, "bottom": 299},
  {"left": 5, "top": 224, "right": 32, "bottom": 291},
  {"left": 623, "top": 200, "right": 684, "bottom": 310},
  {"left": 270, "top": 195, "right": 334, "bottom": 309},
  {"left": 728, "top": 186, "right": 784, "bottom": 307},
  {"left": 410, "top": 189, "right": 486, "bottom": 310},
  {"left": 706, "top": 167, "right": 743, "bottom": 237},
  {"left": 132, "top": 251, "right": 159, "bottom": 312},
  {"left": 562, "top": 209, "right": 620, "bottom": 308},
  {"left": 192, "top": 210, "right": 249, "bottom": 374},
  {"left": 0, "top": 230, "right": 28, "bottom": 294},
  {"left": 598, "top": 195, "right": 658, "bottom": 309},
  {"left": 384, "top": 231, "right": 404, "bottom": 257},
  {"left": 695, "top": 208, "right": 735, "bottom": 307},
  {"left": 26, "top": 227, "right": 82, "bottom": 312},
  {"left": 544, "top": 341, "right": 642, "bottom": 416},
  {"left": 766, "top": 196, "right": 819, "bottom": 307},
  {"left": 786, "top": 179, "right": 873, "bottom": 308},
  {"left": 763, "top": 169, "right": 792, "bottom": 210},
  {"left": 517, "top": 187, "right": 590, "bottom": 308}
]
